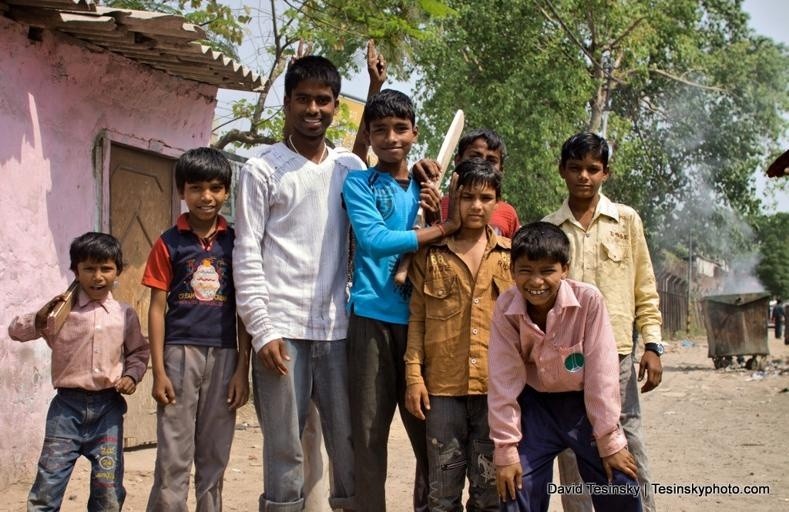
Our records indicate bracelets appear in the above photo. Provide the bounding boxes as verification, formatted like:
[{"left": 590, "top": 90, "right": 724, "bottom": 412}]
[{"left": 436, "top": 222, "right": 447, "bottom": 236}]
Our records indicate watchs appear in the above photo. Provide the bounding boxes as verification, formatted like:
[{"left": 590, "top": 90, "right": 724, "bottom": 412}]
[{"left": 644, "top": 343, "right": 664, "bottom": 356}]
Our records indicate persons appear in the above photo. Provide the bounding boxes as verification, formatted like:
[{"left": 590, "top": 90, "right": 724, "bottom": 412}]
[
  {"left": 230, "top": 55, "right": 369, "bottom": 511},
  {"left": 403, "top": 159, "right": 516, "bottom": 511},
  {"left": 487, "top": 222, "right": 643, "bottom": 511},
  {"left": 8, "top": 231, "right": 150, "bottom": 512},
  {"left": 352, "top": 37, "right": 385, "bottom": 159},
  {"left": 342, "top": 88, "right": 463, "bottom": 511},
  {"left": 411, "top": 130, "right": 522, "bottom": 238},
  {"left": 539, "top": 131, "right": 664, "bottom": 511},
  {"left": 771, "top": 298, "right": 785, "bottom": 338},
  {"left": 142, "top": 146, "right": 253, "bottom": 512}
]
